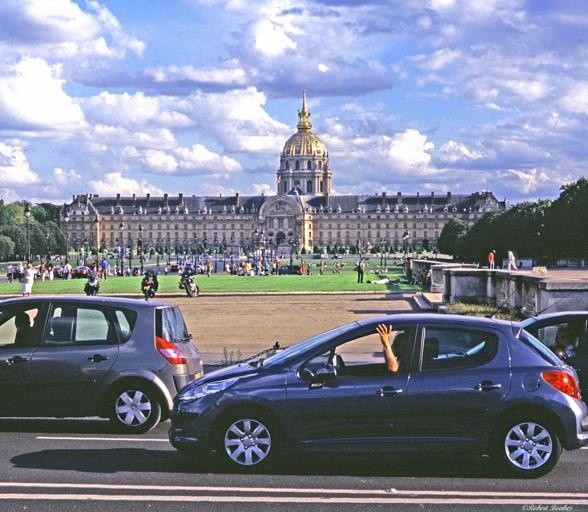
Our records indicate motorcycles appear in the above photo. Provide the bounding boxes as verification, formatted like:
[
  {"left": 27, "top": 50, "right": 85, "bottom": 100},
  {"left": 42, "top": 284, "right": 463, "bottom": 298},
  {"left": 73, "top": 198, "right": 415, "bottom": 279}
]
[
  {"left": 141, "top": 271, "right": 158, "bottom": 299},
  {"left": 180, "top": 273, "right": 200, "bottom": 297},
  {"left": 85, "top": 268, "right": 100, "bottom": 296}
]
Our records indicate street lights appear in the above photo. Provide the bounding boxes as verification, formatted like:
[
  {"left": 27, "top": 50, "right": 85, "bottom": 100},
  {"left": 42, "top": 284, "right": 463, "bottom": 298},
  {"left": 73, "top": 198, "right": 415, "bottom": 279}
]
[
  {"left": 92, "top": 214, "right": 101, "bottom": 270},
  {"left": 76, "top": 239, "right": 79, "bottom": 265},
  {"left": 402, "top": 230, "right": 409, "bottom": 258},
  {"left": 155, "top": 239, "right": 187, "bottom": 266},
  {"left": 139, "top": 225, "right": 144, "bottom": 274},
  {"left": 119, "top": 221, "right": 124, "bottom": 275},
  {"left": 193, "top": 233, "right": 293, "bottom": 276},
  {"left": 24, "top": 203, "right": 31, "bottom": 262},
  {"left": 83, "top": 238, "right": 89, "bottom": 266},
  {"left": 380, "top": 238, "right": 386, "bottom": 265},
  {"left": 63, "top": 210, "right": 69, "bottom": 262}
]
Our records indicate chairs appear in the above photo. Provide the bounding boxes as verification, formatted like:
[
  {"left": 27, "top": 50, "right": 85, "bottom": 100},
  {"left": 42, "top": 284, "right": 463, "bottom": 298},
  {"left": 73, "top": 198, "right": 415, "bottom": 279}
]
[
  {"left": 421, "top": 337, "right": 440, "bottom": 359},
  {"left": 50, "top": 317, "right": 73, "bottom": 342}
]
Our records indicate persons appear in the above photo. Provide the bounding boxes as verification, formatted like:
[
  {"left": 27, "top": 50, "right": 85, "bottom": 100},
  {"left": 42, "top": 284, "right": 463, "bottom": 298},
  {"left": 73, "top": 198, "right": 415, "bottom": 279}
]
[
  {"left": 505, "top": 251, "right": 517, "bottom": 271},
  {"left": 554, "top": 326, "right": 576, "bottom": 359},
  {"left": 376, "top": 323, "right": 404, "bottom": 373},
  {"left": 6, "top": 243, "right": 212, "bottom": 297},
  {"left": 14, "top": 312, "right": 32, "bottom": 343},
  {"left": 488, "top": 249, "right": 496, "bottom": 269},
  {"left": 224, "top": 247, "right": 414, "bottom": 286}
]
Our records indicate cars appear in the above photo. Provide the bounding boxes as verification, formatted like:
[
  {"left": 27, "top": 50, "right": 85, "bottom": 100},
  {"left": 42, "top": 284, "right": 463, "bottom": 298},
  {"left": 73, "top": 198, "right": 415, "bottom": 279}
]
[
  {"left": 171, "top": 262, "right": 206, "bottom": 274},
  {"left": 169, "top": 309, "right": 588, "bottom": 479},
  {"left": 0, "top": 295, "right": 204, "bottom": 432},
  {"left": 432, "top": 309, "right": 588, "bottom": 409},
  {"left": 278, "top": 266, "right": 309, "bottom": 274},
  {"left": 11, "top": 264, "right": 93, "bottom": 278}
]
